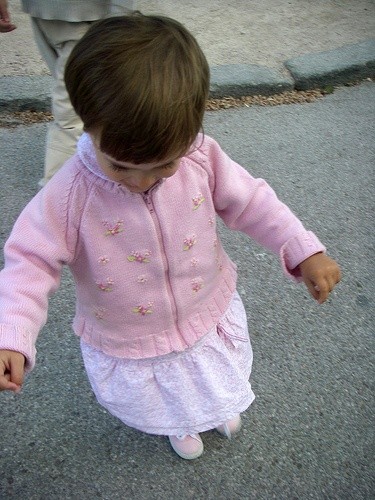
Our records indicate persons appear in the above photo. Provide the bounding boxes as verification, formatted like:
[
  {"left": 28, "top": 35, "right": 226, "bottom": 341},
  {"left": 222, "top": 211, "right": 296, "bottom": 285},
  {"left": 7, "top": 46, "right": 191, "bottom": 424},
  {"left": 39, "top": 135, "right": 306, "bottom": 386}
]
[
  {"left": 0, "top": 0, "right": 182, "bottom": 188},
  {"left": 0, "top": 14, "right": 342, "bottom": 460}
]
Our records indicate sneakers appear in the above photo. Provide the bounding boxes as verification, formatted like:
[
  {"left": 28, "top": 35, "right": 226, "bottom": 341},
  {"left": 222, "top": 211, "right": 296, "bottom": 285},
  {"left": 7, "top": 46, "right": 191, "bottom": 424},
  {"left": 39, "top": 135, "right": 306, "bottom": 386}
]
[
  {"left": 166, "top": 431, "right": 205, "bottom": 460},
  {"left": 216, "top": 407, "right": 243, "bottom": 437}
]
